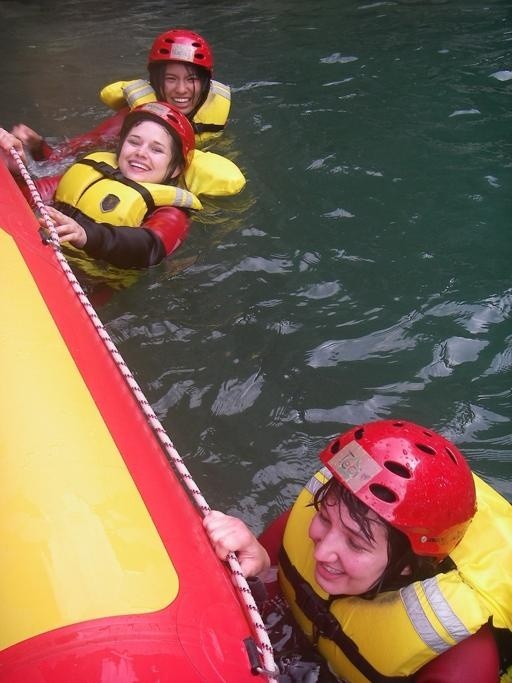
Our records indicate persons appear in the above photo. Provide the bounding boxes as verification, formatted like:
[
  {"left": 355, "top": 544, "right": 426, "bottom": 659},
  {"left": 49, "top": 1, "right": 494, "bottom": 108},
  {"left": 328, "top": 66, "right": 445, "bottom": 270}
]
[
  {"left": 9, "top": 27, "right": 234, "bottom": 170},
  {"left": 1, "top": 100, "right": 205, "bottom": 309},
  {"left": 199, "top": 415, "right": 512, "bottom": 683}
]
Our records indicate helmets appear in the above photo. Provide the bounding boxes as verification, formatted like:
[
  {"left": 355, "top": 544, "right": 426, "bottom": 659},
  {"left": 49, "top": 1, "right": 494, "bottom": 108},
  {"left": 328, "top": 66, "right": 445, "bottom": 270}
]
[
  {"left": 121, "top": 101, "right": 195, "bottom": 175},
  {"left": 148, "top": 29, "right": 214, "bottom": 70},
  {"left": 320, "top": 419, "right": 477, "bottom": 560}
]
